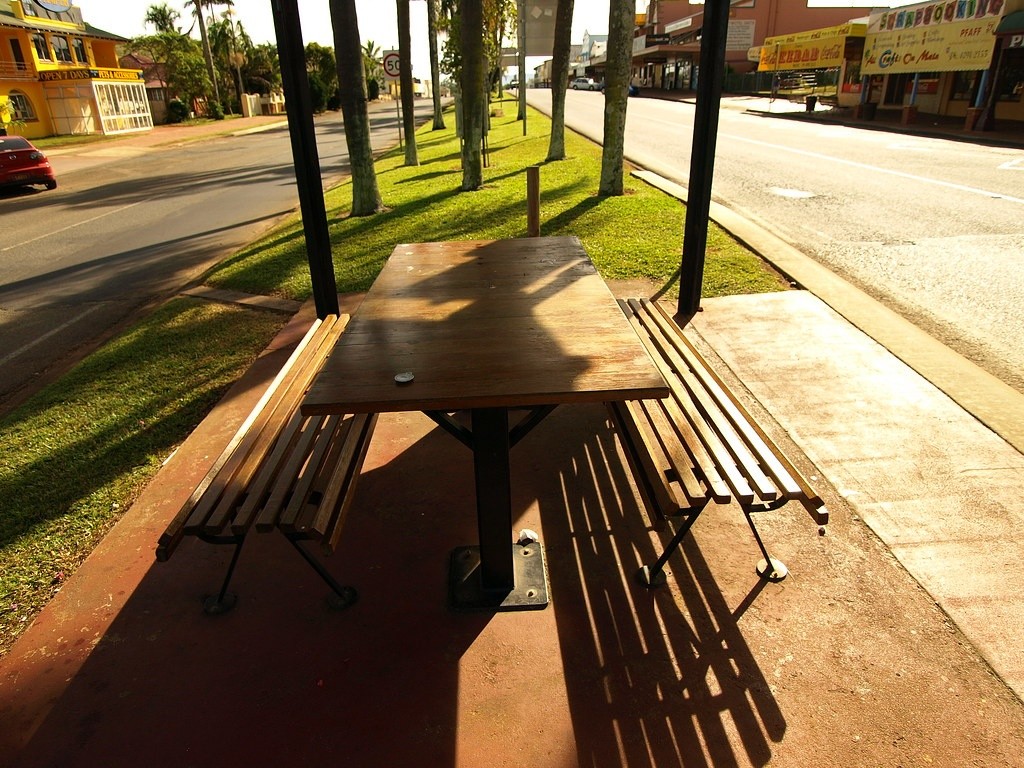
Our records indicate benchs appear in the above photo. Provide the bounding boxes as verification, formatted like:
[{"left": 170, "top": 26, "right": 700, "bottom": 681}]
[
  {"left": 154, "top": 312, "right": 380, "bottom": 612},
  {"left": 604, "top": 299, "right": 830, "bottom": 589}
]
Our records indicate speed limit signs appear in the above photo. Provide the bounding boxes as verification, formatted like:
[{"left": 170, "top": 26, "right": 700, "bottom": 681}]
[{"left": 383, "top": 50, "right": 400, "bottom": 80}]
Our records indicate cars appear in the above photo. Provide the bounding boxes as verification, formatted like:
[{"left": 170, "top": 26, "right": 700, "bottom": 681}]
[
  {"left": 0, "top": 135, "right": 57, "bottom": 190},
  {"left": 510, "top": 80, "right": 519, "bottom": 90},
  {"left": 569, "top": 78, "right": 602, "bottom": 91}
]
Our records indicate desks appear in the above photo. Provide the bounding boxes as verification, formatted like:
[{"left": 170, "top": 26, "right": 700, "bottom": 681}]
[{"left": 299, "top": 236, "right": 670, "bottom": 614}]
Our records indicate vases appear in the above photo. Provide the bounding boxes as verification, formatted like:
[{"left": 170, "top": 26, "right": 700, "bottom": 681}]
[
  {"left": 806, "top": 97, "right": 816, "bottom": 111},
  {"left": 860, "top": 104, "right": 877, "bottom": 120}
]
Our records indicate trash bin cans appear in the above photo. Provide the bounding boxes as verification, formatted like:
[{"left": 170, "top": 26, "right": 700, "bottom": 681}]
[
  {"left": 901, "top": 104, "right": 919, "bottom": 125},
  {"left": 862, "top": 102, "right": 878, "bottom": 120}
]
